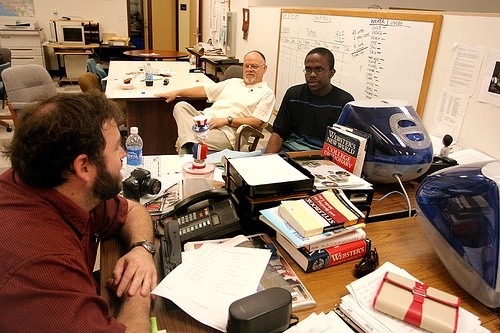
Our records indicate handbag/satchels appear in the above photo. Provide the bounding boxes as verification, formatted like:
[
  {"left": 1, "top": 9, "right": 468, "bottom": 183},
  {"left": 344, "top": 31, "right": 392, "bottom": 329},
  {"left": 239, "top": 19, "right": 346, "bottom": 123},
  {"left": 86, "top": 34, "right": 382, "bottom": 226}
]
[{"left": 373, "top": 272, "right": 461, "bottom": 333}]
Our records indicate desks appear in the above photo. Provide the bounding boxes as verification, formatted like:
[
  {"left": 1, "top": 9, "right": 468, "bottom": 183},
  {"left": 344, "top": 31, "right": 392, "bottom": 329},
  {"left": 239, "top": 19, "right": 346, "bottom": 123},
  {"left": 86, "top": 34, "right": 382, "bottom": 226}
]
[
  {"left": 44, "top": 38, "right": 131, "bottom": 87},
  {"left": 123, "top": 50, "right": 189, "bottom": 61},
  {"left": 99, "top": 151, "right": 500, "bottom": 333},
  {"left": 186, "top": 47, "right": 239, "bottom": 83},
  {"left": 104, "top": 61, "right": 217, "bottom": 155}
]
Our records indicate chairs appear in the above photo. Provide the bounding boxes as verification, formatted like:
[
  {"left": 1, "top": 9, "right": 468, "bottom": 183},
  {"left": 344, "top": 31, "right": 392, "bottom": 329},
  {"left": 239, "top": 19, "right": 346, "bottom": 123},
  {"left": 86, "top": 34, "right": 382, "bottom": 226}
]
[
  {"left": 235, "top": 125, "right": 265, "bottom": 153},
  {"left": 222, "top": 65, "right": 243, "bottom": 81},
  {"left": 1, "top": 64, "right": 82, "bottom": 128},
  {"left": 78, "top": 73, "right": 101, "bottom": 96},
  {"left": 86, "top": 59, "right": 108, "bottom": 88},
  {"left": 0, "top": 47, "right": 12, "bottom": 133}
]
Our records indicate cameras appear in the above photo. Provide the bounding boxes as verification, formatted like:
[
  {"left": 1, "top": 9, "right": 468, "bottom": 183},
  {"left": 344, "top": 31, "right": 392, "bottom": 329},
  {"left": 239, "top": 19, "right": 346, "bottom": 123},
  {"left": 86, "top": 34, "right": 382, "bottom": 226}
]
[{"left": 121, "top": 168, "right": 161, "bottom": 201}]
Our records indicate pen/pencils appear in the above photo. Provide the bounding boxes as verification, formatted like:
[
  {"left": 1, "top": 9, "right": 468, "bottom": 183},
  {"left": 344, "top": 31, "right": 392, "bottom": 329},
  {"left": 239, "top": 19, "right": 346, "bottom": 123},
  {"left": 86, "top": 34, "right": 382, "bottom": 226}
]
[{"left": 144, "top": 191, "right": 172, "bottom": 206}]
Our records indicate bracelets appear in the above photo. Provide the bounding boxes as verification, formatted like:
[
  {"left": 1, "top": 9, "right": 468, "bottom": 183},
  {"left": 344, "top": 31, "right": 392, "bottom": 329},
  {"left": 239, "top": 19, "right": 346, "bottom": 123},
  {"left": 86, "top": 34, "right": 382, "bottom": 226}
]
[{"left": 226, "top": 115, "right": 234, "bottom": 127}]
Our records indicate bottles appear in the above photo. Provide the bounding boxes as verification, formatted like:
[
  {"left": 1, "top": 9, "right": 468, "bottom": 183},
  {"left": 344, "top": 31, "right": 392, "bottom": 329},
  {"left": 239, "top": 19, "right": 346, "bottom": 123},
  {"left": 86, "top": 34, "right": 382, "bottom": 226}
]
[
  {"left": 125, "top": 126, "right": 144, "bottom": 176},
  {"left": 54, "top": 8, "right": 58, "bottom": 20},
  {"left": 145, "top": 63, "right": 153, "bottom": 87}
]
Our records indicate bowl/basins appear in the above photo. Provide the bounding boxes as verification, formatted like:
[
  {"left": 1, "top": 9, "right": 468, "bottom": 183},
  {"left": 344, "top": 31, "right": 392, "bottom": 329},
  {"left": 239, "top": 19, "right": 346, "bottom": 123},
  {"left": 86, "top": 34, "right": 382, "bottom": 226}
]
[{"left": 119, "top": 79, "right": 135, "bottom": 90}]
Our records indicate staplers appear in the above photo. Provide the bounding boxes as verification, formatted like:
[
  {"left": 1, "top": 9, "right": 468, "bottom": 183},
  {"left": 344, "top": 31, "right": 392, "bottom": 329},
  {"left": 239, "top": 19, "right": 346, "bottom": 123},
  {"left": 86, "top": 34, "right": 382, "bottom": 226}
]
[{"left": 163, "top": 79, "right": 169, "bottom": 85}]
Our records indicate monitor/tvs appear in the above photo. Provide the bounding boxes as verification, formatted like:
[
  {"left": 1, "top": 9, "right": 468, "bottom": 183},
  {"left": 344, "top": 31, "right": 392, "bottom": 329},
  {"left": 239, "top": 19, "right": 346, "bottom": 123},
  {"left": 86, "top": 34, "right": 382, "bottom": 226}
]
[{"left": 61, "top": 25, "right": 85, "bottom": 45}]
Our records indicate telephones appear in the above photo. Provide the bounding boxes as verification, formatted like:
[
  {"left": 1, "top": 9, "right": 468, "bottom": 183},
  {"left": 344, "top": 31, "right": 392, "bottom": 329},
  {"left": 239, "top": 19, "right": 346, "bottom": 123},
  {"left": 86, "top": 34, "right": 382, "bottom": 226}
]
[{"left": 173, "top": 188, "right": 242, "bottom": 246}]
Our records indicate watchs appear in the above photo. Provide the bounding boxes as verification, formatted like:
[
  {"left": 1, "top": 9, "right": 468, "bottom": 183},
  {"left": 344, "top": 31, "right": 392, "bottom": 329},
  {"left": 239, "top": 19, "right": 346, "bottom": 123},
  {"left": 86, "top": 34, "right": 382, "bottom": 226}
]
[{"left": 127, "top": 239, "right": 160, "bottom": 255}]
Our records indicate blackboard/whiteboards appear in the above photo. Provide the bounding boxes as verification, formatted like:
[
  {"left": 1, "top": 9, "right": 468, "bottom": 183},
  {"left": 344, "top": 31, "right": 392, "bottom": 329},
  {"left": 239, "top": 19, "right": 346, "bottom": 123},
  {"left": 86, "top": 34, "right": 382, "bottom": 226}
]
[{"left": 273, "top": 7, "right": 444, "bottom": 122}]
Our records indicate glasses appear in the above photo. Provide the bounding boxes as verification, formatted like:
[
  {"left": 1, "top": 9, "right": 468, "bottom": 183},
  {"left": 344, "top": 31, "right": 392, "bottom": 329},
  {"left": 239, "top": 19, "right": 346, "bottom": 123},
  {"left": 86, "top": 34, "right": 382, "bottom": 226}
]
[
  {"left": 243, "top": 64, "right": 263, "bottom": 69},
  {"left": 303, "top": 68, "right": 330, "bottom": 74}
]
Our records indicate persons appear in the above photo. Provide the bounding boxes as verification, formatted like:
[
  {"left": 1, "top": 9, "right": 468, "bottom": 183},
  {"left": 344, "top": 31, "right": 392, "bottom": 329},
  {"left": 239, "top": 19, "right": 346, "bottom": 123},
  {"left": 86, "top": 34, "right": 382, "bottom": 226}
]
[
  {"left": 264, "top": 46, "right": 356, "bottom": 154},
  {"left": 0, "top": 87, "right": 166, "bottom": 333},
  {"left": 172, "top": 49, "right": 277, "bottom": 155}
]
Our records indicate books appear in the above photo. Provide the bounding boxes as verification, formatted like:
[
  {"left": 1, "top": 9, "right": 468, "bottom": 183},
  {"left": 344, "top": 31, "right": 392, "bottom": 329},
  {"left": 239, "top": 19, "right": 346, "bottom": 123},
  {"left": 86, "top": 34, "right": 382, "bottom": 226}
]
[
  {"left": 295, "top": 158, "right": 374, "bottom": 192},
  {"left": 337, "top": 258, "right": 493, "bottom": 333},
  {"left": 258, "top": 186, "right": 373, "bottom": 274},
  {"left": 321, "top": 123, "right": 373, "bottom": 177},
  {"left": 183, "top": 231, "right": 315, "bottom": 318}
]
